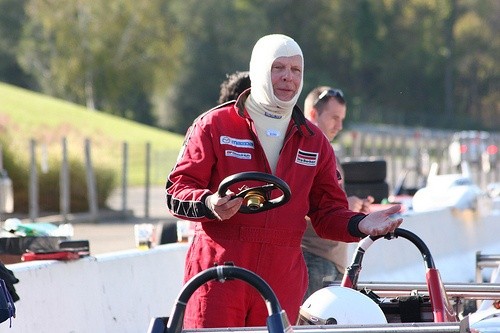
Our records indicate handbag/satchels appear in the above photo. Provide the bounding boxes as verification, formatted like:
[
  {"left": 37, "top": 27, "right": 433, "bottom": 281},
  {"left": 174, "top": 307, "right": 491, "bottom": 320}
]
[{"left": 360, "top": 284, "right": 461, "bottom": 324}]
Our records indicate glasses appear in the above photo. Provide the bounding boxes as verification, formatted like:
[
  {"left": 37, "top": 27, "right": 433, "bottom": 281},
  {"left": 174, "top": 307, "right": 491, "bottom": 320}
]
[{"left": 313, "top": 89, "right": 344, "bottom": 108}]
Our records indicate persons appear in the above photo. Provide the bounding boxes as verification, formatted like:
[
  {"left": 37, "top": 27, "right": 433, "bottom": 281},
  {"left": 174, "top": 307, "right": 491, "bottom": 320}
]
[
  {"left": 164, "top": 34, "right": 404, "bottom": 329},
  {"left": 302, "top": 86, "right": 375, "bottom": 306}
]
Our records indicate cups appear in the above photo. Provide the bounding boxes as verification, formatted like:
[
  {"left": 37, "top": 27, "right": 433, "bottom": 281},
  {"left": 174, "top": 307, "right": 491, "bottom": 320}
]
[
  {"left": 176, "top": 220, "right": 190, "bottom": 242},
  {"left": 135, "top": 224, "right": 153, "bottom": 249}
]
